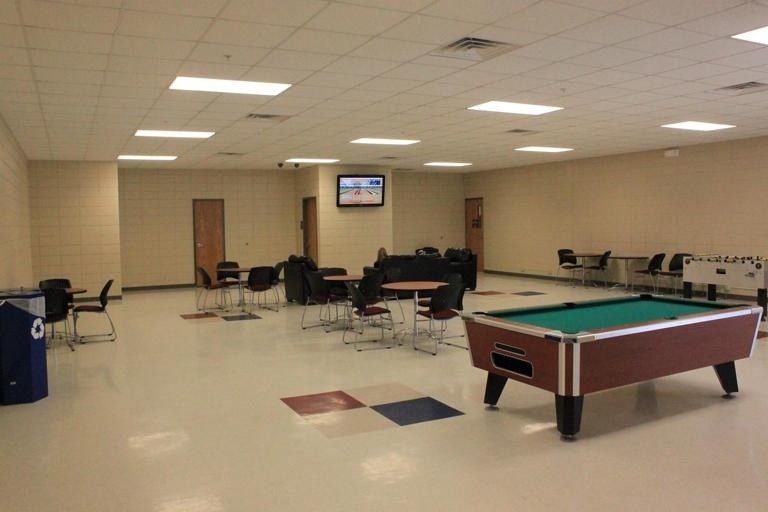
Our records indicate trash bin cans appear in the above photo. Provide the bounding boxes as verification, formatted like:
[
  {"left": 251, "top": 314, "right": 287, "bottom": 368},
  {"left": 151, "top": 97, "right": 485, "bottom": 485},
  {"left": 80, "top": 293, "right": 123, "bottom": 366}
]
[{"left": 0, "top": 301, "right": 48, "bottom": 404}]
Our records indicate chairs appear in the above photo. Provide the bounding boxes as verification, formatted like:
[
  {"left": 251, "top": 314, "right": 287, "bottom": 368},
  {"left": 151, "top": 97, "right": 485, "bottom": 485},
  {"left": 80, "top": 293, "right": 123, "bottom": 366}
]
[
  {"left": 197, "top": 244, "right": 470, "bottom": 368},
  {"left": 43, "top": 289, "right": 71, "bottom": 349},
  {"left": 557, "top": 240, "right": 695, "bottom": 296},
  {"left": 71, "top": 279, "right": 119, "bottom": 342},
  {"left": 39, "top": 278, "right": 71, "bottom": 288}
]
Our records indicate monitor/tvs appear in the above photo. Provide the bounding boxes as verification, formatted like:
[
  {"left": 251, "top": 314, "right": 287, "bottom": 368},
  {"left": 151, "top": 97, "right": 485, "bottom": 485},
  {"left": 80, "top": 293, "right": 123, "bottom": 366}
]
[{"left": 337, "top": 175, "right": 385, "bottom": 207}]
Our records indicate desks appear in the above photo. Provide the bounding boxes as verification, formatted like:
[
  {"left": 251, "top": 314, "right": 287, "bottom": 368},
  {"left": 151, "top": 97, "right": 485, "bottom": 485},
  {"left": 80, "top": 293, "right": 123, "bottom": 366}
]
[
  {"left": 459, "top": 293, "right": 763, "bottom": 442},
  {"left": 0, "top": 288, "right": 88, "bottom": 346}
]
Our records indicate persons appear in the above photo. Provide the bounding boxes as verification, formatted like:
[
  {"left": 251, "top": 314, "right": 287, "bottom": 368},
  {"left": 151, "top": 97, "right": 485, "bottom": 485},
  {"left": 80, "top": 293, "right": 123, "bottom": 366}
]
[{"left": 373, "top": 247, "right": 386, "bottom": 268}]
